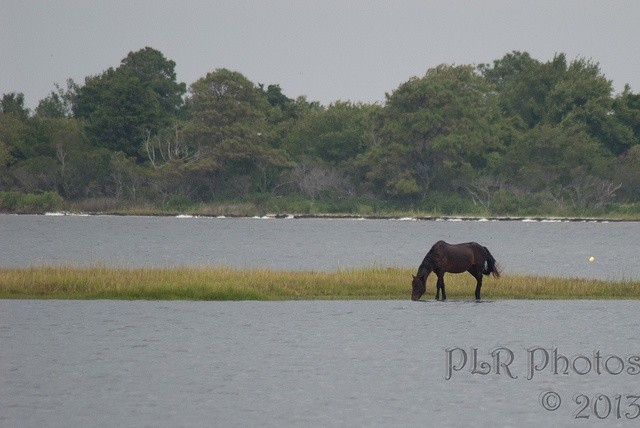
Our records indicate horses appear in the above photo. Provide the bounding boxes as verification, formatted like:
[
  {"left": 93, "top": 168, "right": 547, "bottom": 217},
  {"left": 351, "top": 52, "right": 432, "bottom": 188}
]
[{"left": 411, "top": 240, "right": 501, "bottom": 301}]
